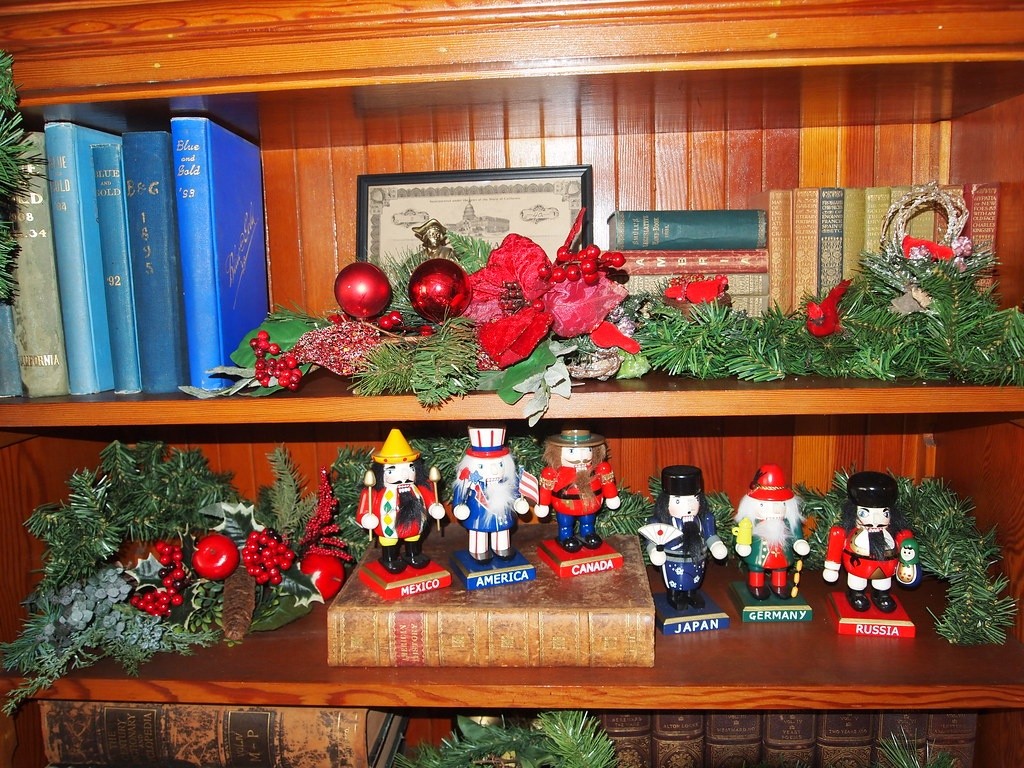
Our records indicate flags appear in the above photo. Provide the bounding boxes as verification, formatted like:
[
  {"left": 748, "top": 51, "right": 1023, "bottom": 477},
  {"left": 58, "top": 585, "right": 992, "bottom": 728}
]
[{"left": 517, "top": 468, "right": 540, "bottom": 504}]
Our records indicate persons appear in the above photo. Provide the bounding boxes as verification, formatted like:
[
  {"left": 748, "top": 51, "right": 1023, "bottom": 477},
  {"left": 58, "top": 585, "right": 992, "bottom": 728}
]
[
  {"left": 452, "top": 424, "right": 530, "bottom": 564},
  {"left": 736, "top": 464, "right": 811, "bottom": 600},
  {"left": 358, "top": 430, "right": 446, "bottom": 571},
  {"left": 823, "top": 471, "right": 919, "bottom": 610},
  {"left": 405, "top": 219, "right": 454, "bottom": 275},
  {"left": 535, "top": 426, "right": 621, "bottom": 552},
  {"left": 645, "top": 464, "right": 728, "bottom": 612}
]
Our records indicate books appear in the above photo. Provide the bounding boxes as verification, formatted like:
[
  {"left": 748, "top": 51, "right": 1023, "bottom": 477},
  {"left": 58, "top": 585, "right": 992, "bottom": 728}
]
[
  {"left": 609, "top": 184, "right": 997, "bottom": 322},
  {"left": 0, "top": 115, "right": 269, "bottom": 398},
  {"left": 326, "top": 521, "right": 655, "bottom": 668},
  {"left": 44, "top": 703, "right": 979, "bottom": 768}
]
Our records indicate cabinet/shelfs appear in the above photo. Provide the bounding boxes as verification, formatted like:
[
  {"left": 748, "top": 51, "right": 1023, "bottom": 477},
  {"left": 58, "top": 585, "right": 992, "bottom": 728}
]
[{"left": 0, "top": 0, "right": 1024, "bottom": 768}]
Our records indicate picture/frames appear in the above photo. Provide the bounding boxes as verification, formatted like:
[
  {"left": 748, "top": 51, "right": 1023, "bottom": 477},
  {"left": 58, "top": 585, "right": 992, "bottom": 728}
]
[{"left": 354, "top": 163, "right": 595, "bottom": 270}]
